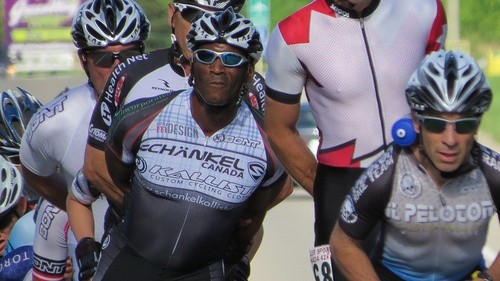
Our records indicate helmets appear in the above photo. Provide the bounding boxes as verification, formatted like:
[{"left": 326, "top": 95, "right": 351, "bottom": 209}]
[
  {"left": 174, "top": 0, "right": 245, "bottom": 13},
  {"left": 405, "top": 50, "right": 492, "bottom": 118},
  {"left": 0, "top": 155, "right": 22, "bottom": 213},
  {"left": 70, "top": 0, "right": 151, "bottom": 47},
  {"left": 0, "top": 87, "right": 43, "bottom": 152},
  {"left": 186, "top": 6, "right": 263, "bottom": 64}
]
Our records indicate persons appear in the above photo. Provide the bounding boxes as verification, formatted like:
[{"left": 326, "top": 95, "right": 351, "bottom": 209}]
[
  {"left": 0, "top": 0, "right": 500, "bottom": 281},
  {"left": 329, "top": 49, "right": 500, "bottom": 281}
]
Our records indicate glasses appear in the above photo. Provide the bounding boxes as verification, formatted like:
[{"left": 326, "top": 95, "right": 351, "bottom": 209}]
[
  {"left": 414, "top": 111, "right": 480, "bottom": 133},
  {"left": 193, "top": 48, "right": 250, "bottom": 67},
  {"left": 173, "top": 3, "right": 208, "bottom": 24},
  {"left": 82, "top": 44, "right": 145, "bottom": 67},
  {"left": 2, "top": 153, "right": 22, "bottom": 166},
  {"left": 0, "top": 205, "right": 19, "bottom": 230}
]
